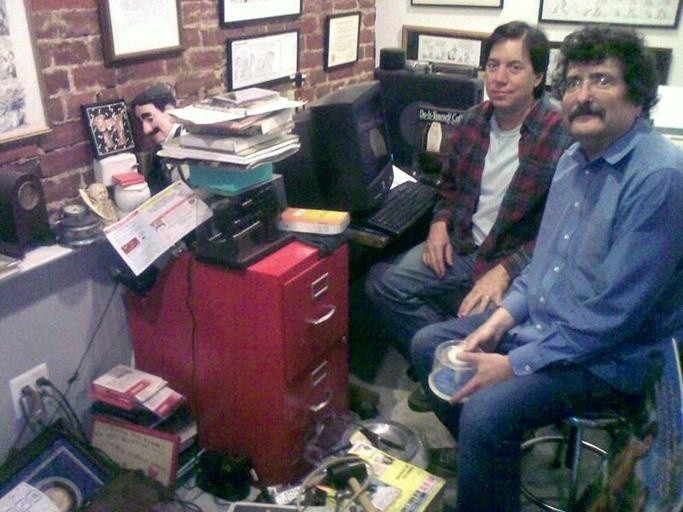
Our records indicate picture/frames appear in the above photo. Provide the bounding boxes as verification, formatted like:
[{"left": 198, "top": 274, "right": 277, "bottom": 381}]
[
  {"left": 323, "top": 11, "right": 361, "bottom": 72},
  {"left": 97, "top": 0, "right": 190, "bottom": 68},
  {"left": 79, "top": 97, "right": 137, "bottom": 161},
  {"left": 401, "top": 24, "right": 493, "bottom": 71},
  {"left": 538, "top": 0, "right": 683, "bottom": 28},
  {"left": 218, "top": 0, "right": 303, "bottom": 28},
  {"left": 544, "top": 41, "right": 565, "bottom": 91},
  {"left": 0, "top": 0, "right": 56, "bottom": 144},
  {"left": 226, "top": 30, "right": 301, "bottom": 90},
  {"left": 410, "top": 0, "right": 503, "bottom": 8}
]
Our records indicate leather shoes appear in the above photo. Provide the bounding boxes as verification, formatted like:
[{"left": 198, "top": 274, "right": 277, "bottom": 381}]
[{"left": 429, "top": 447, "right": 458, "bottom": 474}]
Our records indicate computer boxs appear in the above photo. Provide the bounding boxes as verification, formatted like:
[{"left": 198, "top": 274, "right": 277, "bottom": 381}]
[{"left": 374, "top": 64, "right": 485, "bottom": 164}]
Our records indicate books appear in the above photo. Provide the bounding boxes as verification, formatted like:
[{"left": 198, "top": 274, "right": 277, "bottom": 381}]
[
  {"left": 156, "top": 87, "right": 301, "bottom": 196},
  {"left": 92, "top": 365, "right": 206, "bottom": 479},
  {"left": 315, "top": 442, "right": 447, "bottom": 512},
  {"left": 279, "top": 206, "right": 349, "bottom": 236}
]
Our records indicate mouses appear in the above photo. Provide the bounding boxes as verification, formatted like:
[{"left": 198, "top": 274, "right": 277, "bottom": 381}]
[{"left": 419, "top": 176, "right": 442, "bottom": 187}]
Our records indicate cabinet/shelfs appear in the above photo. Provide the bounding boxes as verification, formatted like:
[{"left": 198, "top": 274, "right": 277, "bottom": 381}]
[{"left": 124, "top": 239, "right": 351, "bottom": 488}]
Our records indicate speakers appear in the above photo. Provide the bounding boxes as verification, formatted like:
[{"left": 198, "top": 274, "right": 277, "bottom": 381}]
[{"left": 0, "top": 169, "right": 55, "bottom": 259}]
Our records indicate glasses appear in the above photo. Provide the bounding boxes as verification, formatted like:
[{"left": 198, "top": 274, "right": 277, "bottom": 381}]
[{"left": 564, "top": 74, "right": 615, "bottom": 90}]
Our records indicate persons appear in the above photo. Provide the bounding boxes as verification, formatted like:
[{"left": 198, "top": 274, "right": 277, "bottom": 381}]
[
  {"left": 131, "top": 88, "right": 188, "bottom": 184},
  {"left": 365, "top": 22, "right": 574, "bottom": 414},
  {"left": 409, "top": 27, "right": 683, "bottom": 512}
]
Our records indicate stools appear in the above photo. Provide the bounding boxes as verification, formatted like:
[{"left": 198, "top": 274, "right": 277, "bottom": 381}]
[{"left": 520, "top": 410, "right": 648, "bottom": 510}]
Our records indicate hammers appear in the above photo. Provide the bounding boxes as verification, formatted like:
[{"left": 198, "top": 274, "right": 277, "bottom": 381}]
[{"left": 326, "top": 459, "right": 376, "bottom": 512}]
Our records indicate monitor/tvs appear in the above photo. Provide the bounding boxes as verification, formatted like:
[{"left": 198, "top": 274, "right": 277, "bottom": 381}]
[{"left": 309, "top": 79, "right": 395, "bottom": 214}]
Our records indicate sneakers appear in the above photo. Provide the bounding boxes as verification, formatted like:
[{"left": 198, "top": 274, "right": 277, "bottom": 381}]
[{"left": 408, "top": 387, "right": 436, "bottom": 412}]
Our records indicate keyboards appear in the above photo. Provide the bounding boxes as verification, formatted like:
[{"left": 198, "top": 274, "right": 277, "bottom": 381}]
[{"left": 356, "top": 181, "right": 439, "bottom": 238}]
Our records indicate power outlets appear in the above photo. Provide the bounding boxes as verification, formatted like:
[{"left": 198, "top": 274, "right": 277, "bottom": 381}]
[{"left": 10, "top": 363, "right": 53, "bottom": 420}]
